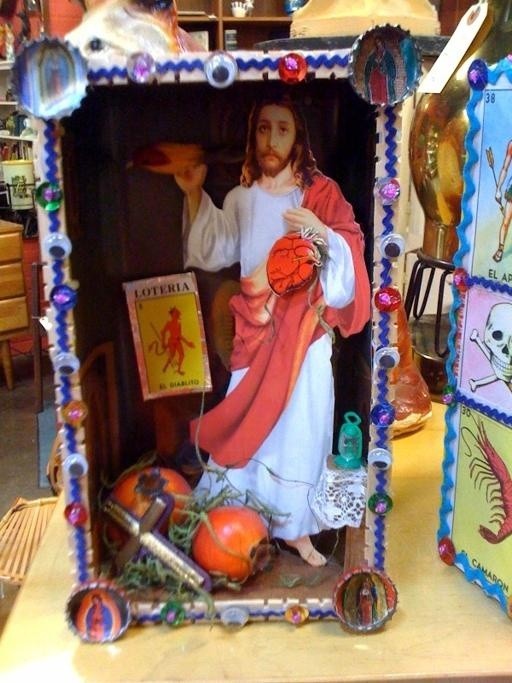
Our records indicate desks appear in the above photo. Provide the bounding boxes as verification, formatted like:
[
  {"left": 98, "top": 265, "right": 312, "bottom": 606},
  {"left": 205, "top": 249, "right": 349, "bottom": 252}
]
[{"left": 0, "top": 403, "right": 512, "bottom": 683}]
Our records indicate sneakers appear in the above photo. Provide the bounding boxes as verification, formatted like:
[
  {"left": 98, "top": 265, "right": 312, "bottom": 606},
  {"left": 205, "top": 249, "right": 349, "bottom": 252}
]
[{"left": 493, "top": 248, "right": 503, "bottom": 262}]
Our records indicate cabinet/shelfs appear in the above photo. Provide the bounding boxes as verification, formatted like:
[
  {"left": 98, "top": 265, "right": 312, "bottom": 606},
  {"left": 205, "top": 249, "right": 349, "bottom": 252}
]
[
  {"left": 0, "top": 220, "right": 29, "bottom": 390},
  {"left": 177, "top": 0, "right": 293, "bottom": 50},
  {"left": 0, "top": 60, "right": 35, "bottom": 142}
]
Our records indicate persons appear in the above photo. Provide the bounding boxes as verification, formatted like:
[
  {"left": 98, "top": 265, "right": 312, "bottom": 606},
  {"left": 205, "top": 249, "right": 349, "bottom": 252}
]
[
  {"left": 492, "top": 140, "right": 512, "bottom": 264},
  {"left": 176, "top": 92, "right": 370, "bottom": 568}
]
